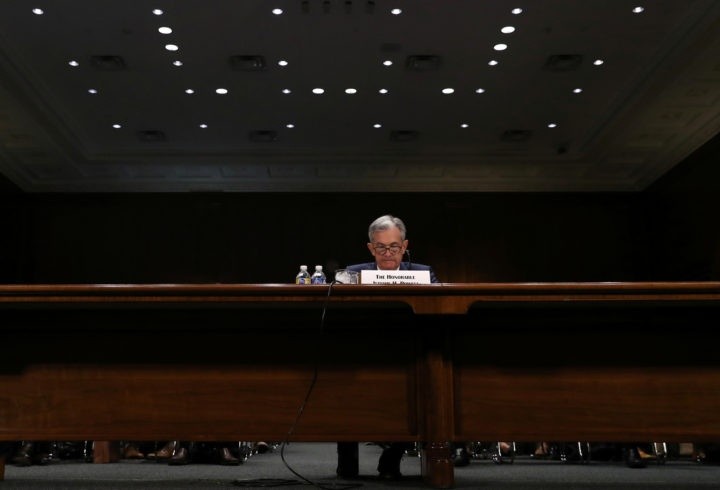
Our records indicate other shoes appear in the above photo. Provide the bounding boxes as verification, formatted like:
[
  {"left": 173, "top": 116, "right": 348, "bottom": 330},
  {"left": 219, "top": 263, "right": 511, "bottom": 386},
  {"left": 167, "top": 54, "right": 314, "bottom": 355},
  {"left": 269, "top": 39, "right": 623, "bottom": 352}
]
[
  {"left": 379, "top": 470, "right": 403, "bottom": 482},
  {"left": 147, "top": 446, "right": 174, "bottom": 458},
  {"left": 219, "top": 448, "right": 240, "bottom": 464},
  {"left": 531, "top": 449, "right": 550, "bottom": 458},
  {"left": 336, "top": 468, "right": 359, "bottom": 480},
  {"left": 257, "top": 445, "right": 269, "bottom": 453},
  {"left": 456, "top": 456, "right": 470, "bottom": 466},
  {"left": 168, "top": 449, "right": 189, "bottom": 465},
  {"left": 626, "top": 449, "right": 647, "bottom": 469},
  {"left": 126, "top": 446, "right": 144, "bottom": 459}
]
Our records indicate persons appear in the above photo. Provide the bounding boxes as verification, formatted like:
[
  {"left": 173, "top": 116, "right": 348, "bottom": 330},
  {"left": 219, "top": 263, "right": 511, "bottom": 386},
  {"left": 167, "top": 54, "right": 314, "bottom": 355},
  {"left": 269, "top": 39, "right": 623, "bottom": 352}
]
[
  {"left": 344, "top": 214, "right": 439, "bottom": 482},
  {"left": 0, "top": 441, "right": 273, "bottom": 466},
  {"left": 401, "top": 438, "right": 720, "bottom": 470}
]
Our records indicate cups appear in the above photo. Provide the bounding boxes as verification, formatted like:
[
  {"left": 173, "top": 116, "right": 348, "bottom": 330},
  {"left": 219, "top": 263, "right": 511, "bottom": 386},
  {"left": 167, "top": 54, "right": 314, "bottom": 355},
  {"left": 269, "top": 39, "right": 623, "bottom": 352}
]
[{"left": 334, "top": 269, "right": 359, "bottom": 284}]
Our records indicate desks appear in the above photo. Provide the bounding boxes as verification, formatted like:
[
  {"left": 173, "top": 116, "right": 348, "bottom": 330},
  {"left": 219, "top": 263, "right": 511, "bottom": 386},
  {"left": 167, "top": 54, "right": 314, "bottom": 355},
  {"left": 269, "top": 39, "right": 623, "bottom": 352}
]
[{"left": 0, "top": 280, "right": 720, "bottom": 490}]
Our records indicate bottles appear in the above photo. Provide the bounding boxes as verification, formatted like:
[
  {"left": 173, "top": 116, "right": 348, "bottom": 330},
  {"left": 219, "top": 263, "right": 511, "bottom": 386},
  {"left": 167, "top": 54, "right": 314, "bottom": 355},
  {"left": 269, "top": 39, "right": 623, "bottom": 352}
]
[
  {"left": 296, "top": 265, "right": 311, "bottom": 284},
  {"left": 311, "top": 265, "right": 326, "bottom": 284}
]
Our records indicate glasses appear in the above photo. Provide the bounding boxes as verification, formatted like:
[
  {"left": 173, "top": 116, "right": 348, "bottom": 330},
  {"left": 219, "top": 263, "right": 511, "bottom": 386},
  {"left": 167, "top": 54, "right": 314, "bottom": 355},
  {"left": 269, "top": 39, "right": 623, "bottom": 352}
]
[{"left": 374, "top": 245, "right": 403, "bottom": 255}]
